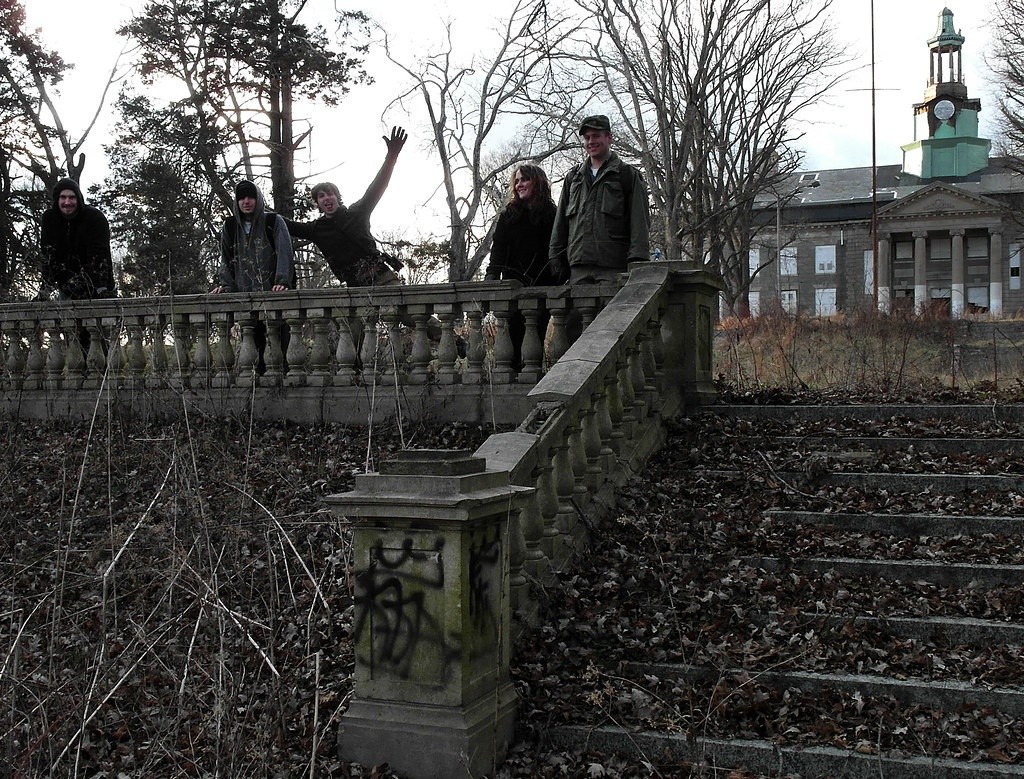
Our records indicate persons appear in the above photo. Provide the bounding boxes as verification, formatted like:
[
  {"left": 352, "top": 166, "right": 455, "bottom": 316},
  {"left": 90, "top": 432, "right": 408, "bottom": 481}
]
[
  {"left": 485, "top": 163, "right": 558, "bottom": 377},
  {"left": 548, "top": 114, "right": 651, "bottom": 347},
  {"left": 208, "top": 179, "right": 298, "bottom": 378},
  {"left": 279, "top": 125, "right": 487, "bottom": 377},
  {"left": 41, "top": 178, "right": 117, "bottom": 371}
]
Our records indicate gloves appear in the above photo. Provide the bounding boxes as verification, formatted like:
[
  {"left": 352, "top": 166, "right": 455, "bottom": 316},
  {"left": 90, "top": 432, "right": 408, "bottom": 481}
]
[
  {"left": 555, "top": 259, "right": 570, "bottom": 284},
  {"left": 97, "top": 289, "right": 118, "bottom": 298},
  {"left": 485, "top": 274, "right": 499, "bottom": 280},
  {"left": 550, "top": 259, "right": 560, "bottom": 276}
]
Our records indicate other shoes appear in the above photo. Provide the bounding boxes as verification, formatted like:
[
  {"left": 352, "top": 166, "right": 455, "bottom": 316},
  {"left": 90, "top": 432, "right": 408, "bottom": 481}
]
[
  {"left": 454, "top": 336, "right": 469, "bottom": 359},
  {"left": 31, "top": 297, "right": 43, "bottom": 302}
]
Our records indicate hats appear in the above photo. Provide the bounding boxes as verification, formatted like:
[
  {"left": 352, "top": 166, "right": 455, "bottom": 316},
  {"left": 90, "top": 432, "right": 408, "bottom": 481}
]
[
  {"left": 236, "top": 181, "right": 256, "bottom": 199},
  {"left": 579, "top": 115, "right": 610, "bottom": 135}
]
